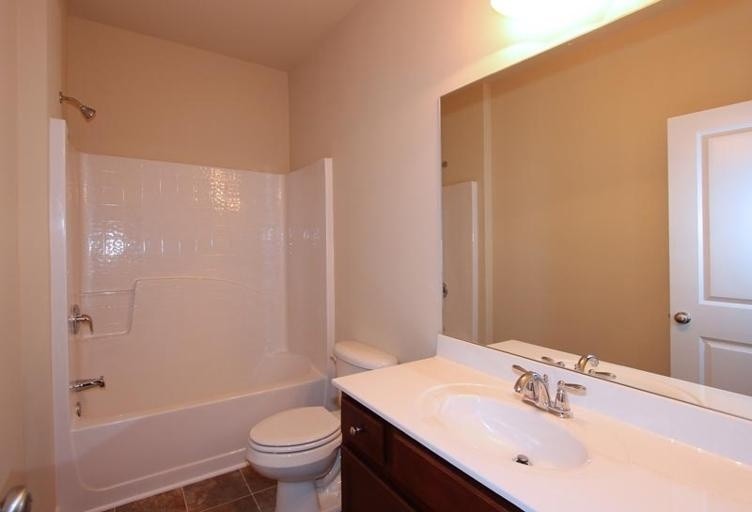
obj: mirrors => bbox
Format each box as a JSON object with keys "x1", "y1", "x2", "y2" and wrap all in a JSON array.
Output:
[{"x1": 439, "y1": 0, "x2": 752, "y2": 423}]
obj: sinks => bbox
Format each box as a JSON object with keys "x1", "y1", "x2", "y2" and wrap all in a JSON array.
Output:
[
  {"x1": 418, "y1": 382, "x2": 590, "y2": 471},
  {"x1": 560, "y1": 361, "x2": 702, "y2": 406}
]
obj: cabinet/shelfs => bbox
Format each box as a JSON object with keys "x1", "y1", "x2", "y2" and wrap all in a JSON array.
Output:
[{"x1": 341, "y1": 391, "x2": 527, "y2": 512}]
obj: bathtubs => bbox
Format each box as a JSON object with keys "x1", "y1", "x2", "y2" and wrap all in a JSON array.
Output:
[{"x1": 70, "y1": 353, "x2": 327, "y2": 511}]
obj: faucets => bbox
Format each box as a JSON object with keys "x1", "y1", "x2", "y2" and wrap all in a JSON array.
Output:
[
  {"x1": 574, "y1": 354, "x2": 599, "y2": 373},
  {"x1": 75, "y1": 376, "x2": 105, "y2": 392},
  {"x1": 514, "y1": 372, "x2": 550, "y2": 409}
]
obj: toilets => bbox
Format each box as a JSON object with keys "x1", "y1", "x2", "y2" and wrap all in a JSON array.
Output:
[{"x1": 244, "y1": 338, "x2": 399, "y2": 511}]
